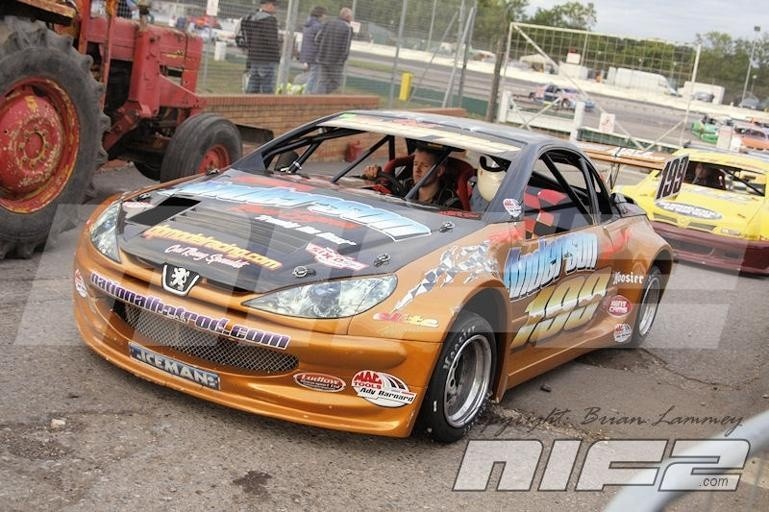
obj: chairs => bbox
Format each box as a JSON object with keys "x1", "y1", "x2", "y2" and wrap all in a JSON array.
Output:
[
  {"x1": 380, "y1": 156, "x2": 474, "y2": 211},
  {"x1": 693, "y1": 167, "x2": 725, "y2": 187},
  {"x1": 517, "y1": 186, "x2": 576, "y2": 240}
]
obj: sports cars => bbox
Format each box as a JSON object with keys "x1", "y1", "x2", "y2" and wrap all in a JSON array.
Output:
[
  {"x1": 71, "y1": 107, "x2": 674, "y2": 446},
  {"x1": 529, "y1": 82, "x2": 595, "y2": 113},
  {"x1": 692, "y1": 113, "x2": 768, "y2": 153},
  {"x1": 607, "y1": 148, "x2": 768, "y2": 278}
]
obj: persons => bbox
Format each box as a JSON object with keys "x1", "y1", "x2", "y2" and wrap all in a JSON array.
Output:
[
  {"x1": 246, "y1": 0, "x2": 281, "y2": 93},
  {"x1": 300, "y1": 5, "x2": 328, "y2": 94},
  {"x1": 363, "y1": 145, "x2": 464, "y2": 210},
  {"x1": 313, "y1": 7, "x2": 353, "y2": 95},
  {"x1": 685, "y1": 163, "x2": 717, "y2": 187}
]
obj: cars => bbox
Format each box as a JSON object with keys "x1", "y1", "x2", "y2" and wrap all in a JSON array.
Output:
[
  {"x1": 691, "y1": 92, "x2": 714, "y2": 104},
  {"x1": 739, "y1": 96, "x2": 768, "y2": 111}
]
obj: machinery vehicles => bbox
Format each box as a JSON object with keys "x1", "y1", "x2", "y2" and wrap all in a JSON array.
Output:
[{"x1": 0, "y1": 0, "x2": 244, "y2": 268}]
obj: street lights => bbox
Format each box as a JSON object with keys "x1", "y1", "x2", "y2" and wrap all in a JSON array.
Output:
[{"x1": 740, "y1": 25, "x2": 763, "y2": 101}]
